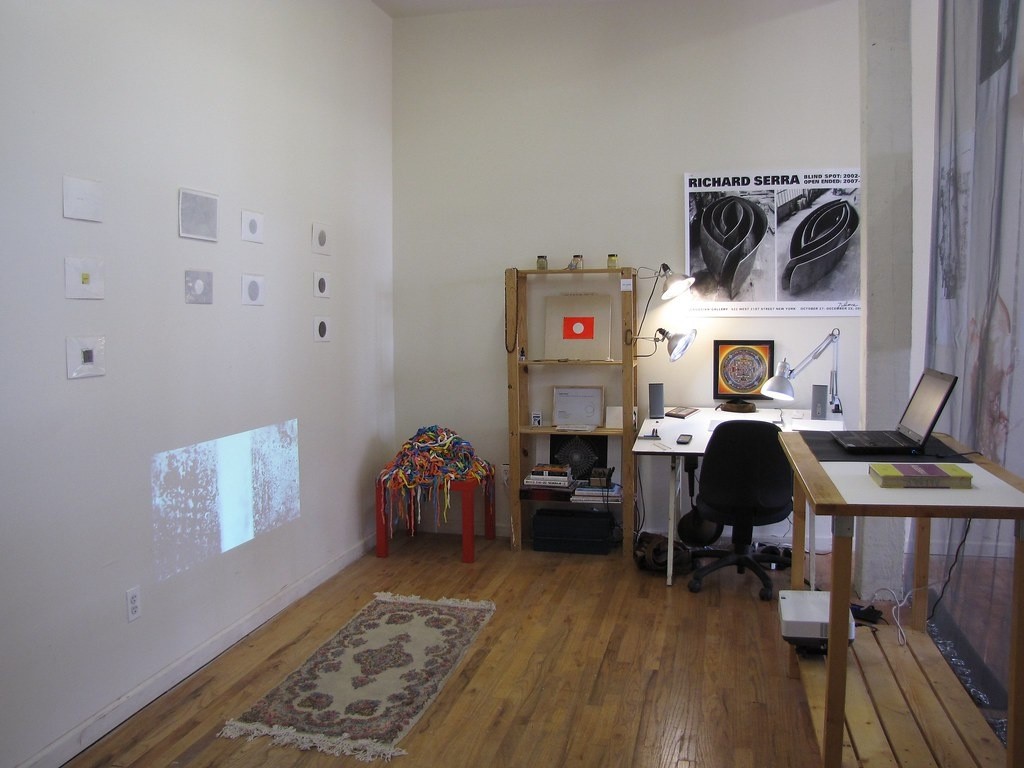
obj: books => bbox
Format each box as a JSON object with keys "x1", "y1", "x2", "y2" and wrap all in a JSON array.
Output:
[
  {"x1": 571, "y1": 477, "x2": 623, "y2": 504},
  {"x1": 868, "y1": 462, "x2": 973, "y2": 489},
  {"x1": 524, "y1": 462, "x2": 576, "y2": 489}
]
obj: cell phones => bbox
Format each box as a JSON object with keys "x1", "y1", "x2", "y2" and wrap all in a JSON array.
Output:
[{"x1": 677, "y1": 434, "x2": 692, "y2": 445}]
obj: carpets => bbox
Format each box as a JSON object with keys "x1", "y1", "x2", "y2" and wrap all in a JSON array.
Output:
[{"x1": 218, "y1": 590, "x2": 495, "y2": 762}]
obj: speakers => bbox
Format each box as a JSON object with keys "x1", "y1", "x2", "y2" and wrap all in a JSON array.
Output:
[
  {"x1": 811, "y1": 385, "x2": 828, "y2": 420},
  {"x1": 649, "y1": 383, "x2": 664, "y2": 419}
]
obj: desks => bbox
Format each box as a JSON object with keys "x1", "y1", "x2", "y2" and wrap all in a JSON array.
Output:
[
  {"x1": 631, "y1": 406, "x2": 844, "y2": 586},
  {"x1": 778, "y1": 431, "x2": 1024, "y2": 768}
]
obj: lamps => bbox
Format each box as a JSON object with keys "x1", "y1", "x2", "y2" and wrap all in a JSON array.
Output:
[
  {"x1": 635, "y1": 327, "x2": 701, "y2": 362},
  {"x1": 638, "y1": 262, "x2": 698, "y2": 338},
  {"x1": 762, "y1": 327, "x2": 842, "y2": 415}
]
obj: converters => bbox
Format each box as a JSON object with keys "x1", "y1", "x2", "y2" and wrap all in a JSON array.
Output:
[{"x1": 850, "y1": 604, "x2": 883, "y2": 624}]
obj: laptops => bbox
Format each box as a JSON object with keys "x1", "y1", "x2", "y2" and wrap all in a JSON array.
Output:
[{"x1": 830, "y1": 367, "x2": 958, "y2": 453}]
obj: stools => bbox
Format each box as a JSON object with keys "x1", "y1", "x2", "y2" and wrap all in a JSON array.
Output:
[{"x1": 375, "y1": 465, "x2": 497, "y2": 564}]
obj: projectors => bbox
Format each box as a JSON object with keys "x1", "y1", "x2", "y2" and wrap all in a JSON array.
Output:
[{"x1": 777, "y1": 590, "x2": 855, "y2": 645}]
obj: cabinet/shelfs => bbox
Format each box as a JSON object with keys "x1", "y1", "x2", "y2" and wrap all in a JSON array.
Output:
[{"x1": 505, "y1": 267, "x2": 637, "y2": 559}]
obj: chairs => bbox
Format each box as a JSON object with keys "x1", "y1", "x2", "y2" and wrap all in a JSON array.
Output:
[{"x1": 687, "y1": 420, "x2": 794, "y2": 600}]
